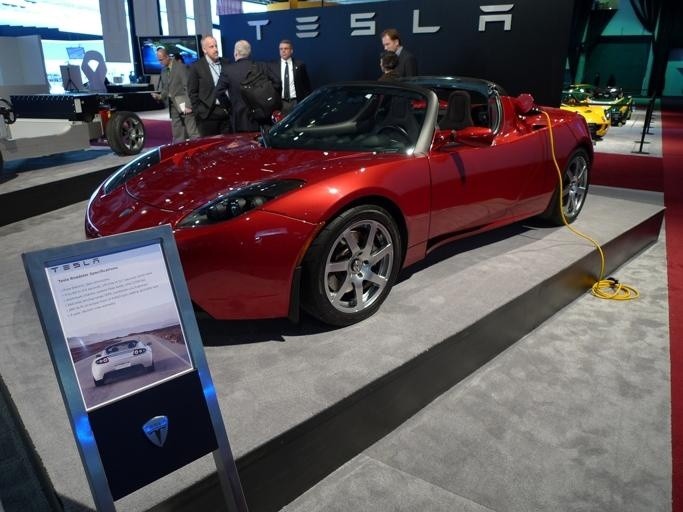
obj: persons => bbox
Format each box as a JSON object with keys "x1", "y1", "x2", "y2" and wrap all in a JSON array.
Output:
[
  {"x1": 377, "y1": 28, "x2": 416, "y2": 81},
  {"x1": 150, "y1": 34, "x2": 311, "y2": 144}
]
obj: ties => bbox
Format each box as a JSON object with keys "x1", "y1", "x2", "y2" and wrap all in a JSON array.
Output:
[{"x1": 284, "y1": 62, "x2": 290, "y2": 101}]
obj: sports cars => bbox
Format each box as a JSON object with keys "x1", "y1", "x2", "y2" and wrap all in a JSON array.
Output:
[
  {"x1": 89, "y1": 339, "x2": 154, "y2": 387},
  {"x1": 85, "y1": 76, "x2": 594, "y2": 327},
  {"x1": 559, "y1": 83, "x2": 633, "y2": 140}
]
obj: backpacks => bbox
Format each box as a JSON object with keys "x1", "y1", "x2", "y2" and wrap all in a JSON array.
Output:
[{"x1": 239, "y1": 60, "x2": 283, "y2": 125}]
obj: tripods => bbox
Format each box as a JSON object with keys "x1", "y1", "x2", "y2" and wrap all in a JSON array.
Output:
[{"x1": 65, "y1": 68, "x2": 79, "y2": 92}]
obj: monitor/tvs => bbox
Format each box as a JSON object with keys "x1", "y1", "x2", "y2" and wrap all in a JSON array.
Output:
[{"x1": 137, "y1": 34, "x2": 200, "y2": 76}]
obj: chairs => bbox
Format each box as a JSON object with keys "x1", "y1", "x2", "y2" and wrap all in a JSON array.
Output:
[{"x1": 366, "y1": 91, "x2": 476, "y2": 144}]
